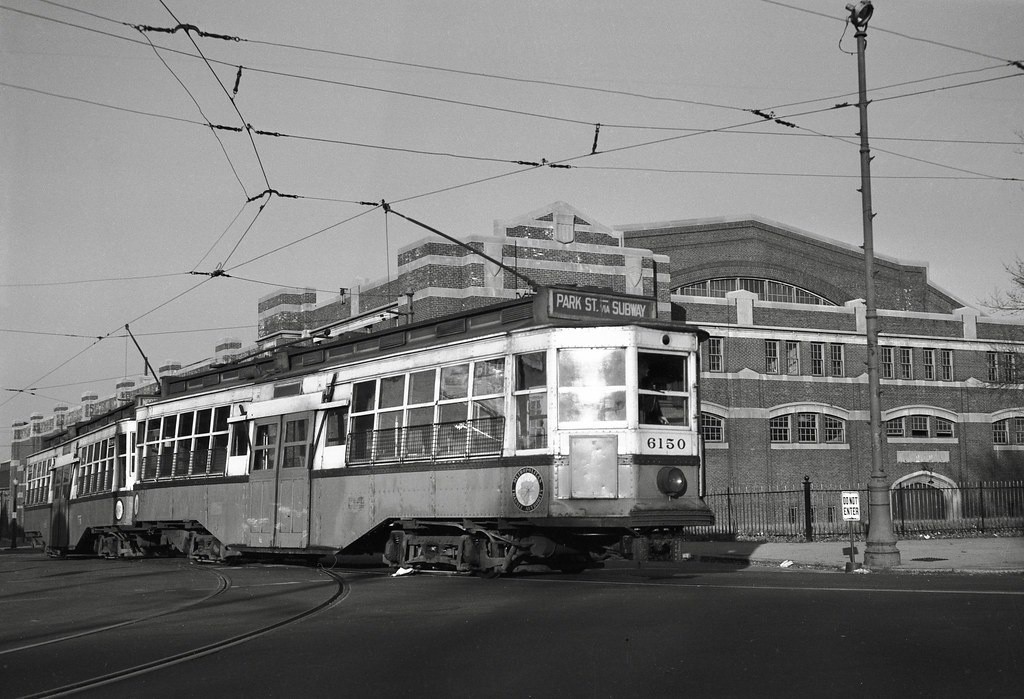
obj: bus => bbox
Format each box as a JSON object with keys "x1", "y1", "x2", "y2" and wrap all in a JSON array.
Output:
[{"x1": 23, "y1": 197, "x2": 716, "y2": 581}]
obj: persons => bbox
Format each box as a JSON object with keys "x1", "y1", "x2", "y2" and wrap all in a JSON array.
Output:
[{"x1": 638, "y1": 359, "x2": 669, "y2": 424}]
